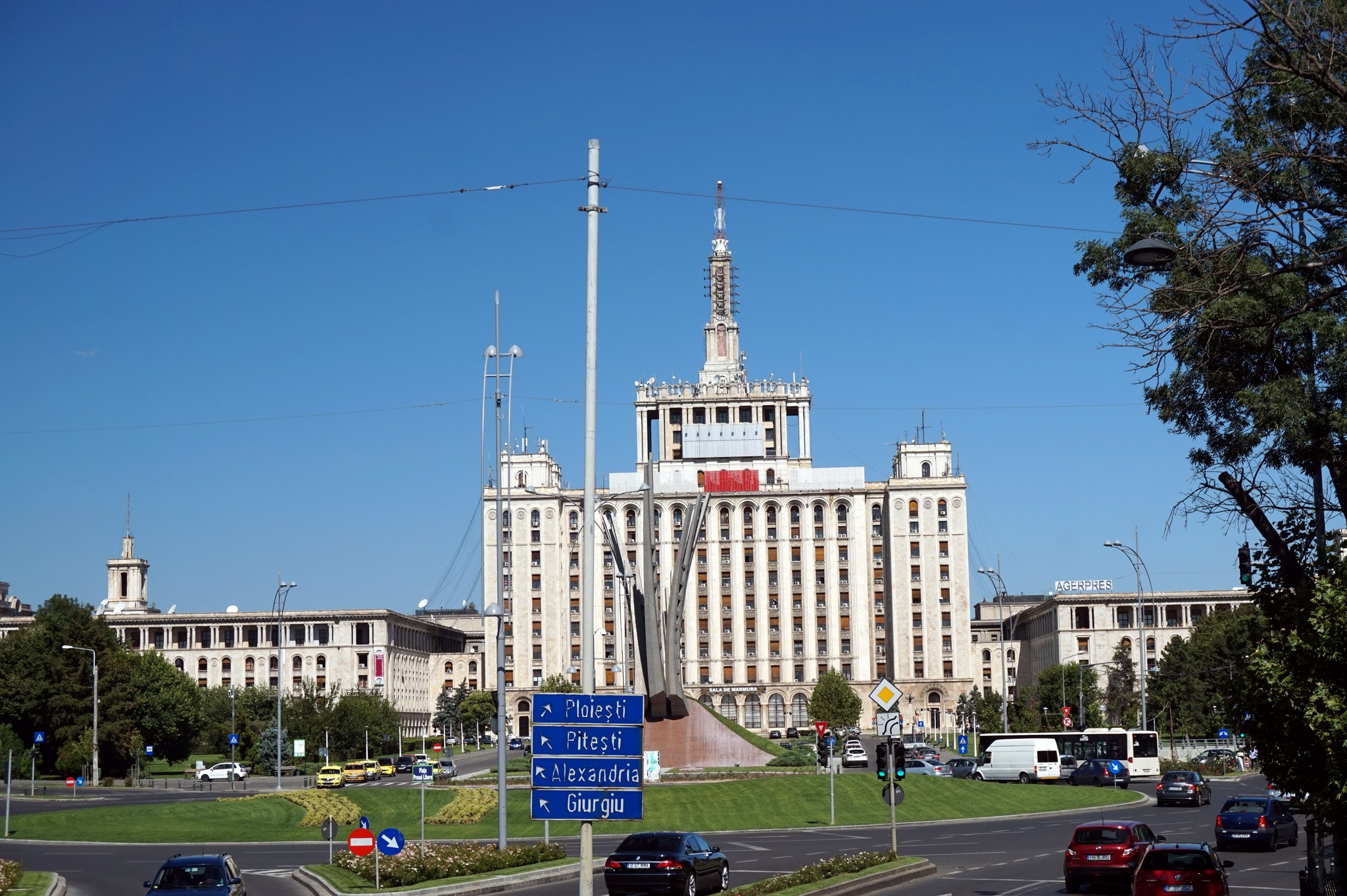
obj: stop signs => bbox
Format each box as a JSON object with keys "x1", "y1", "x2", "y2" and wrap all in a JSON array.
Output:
[
  {"x1": 66, "y1": 777, "x2": 75, "y2": 787},
  {"x1": 433, "y1": 744, "x2": 442, "y2": 752},
  {"x1": 348, "y1": 829, "x2": 376, "y2": 856}
]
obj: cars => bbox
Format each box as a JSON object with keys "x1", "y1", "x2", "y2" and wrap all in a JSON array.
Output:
[
  {"x1": 764, "y1": 725, "x2": 978, "y2": 781},
  {"x1": 1191, "y1": 749, "x2": 1240, "y2": 770},
  {"x1": 414, "y1": 733, "x2": 532, "y2": 757},
  {"x1": 1156, "y1": 770, "x2": 1210, "y2": 807},
  {"x1": 342, "y1": 763, "x2": 368, "y2": 783},
  {"x1": 358, "y1": 753, "x2": 461, "y2": 781},
  {"x1": 603, "y1": 829, "x2": 730, "y2": 896},
  {"x1": 316, "y1": 766, "x2": 346, "y2": 788},
  {"x1": 1069, "y1": 760, "x2": 1130, "y2": 790}
]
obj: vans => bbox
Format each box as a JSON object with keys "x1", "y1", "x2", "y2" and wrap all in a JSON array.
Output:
[
  {"x1": 971, "y1": 738, "x2": 1062, "y2": 786},
  {"x1": 1052, "y1": 754, "x2": 1078, "y2": 784}
]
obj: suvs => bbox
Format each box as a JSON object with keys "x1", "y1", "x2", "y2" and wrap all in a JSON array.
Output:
[
  {"x1": 1214, "y1": 794, "x2": 1299, "y2": 853},
  {"x1": 196, "y1": 762, "x2": 248, "y2": 783},
  {"x1": 1064, "y1": 821, "x2": 1167, "y2": 892},
  {"x1": 1131, "y1": 838, "x2": 1235, "y2": 896},
  {"x1": 1267, "y1": 779, "x2": 1322, "y2": 812},
  {"x1": 142, "y1": 853, "x2": 248, "y2": 896}
]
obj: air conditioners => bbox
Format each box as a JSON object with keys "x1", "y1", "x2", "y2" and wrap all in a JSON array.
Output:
[
  {"x1": 445, "y1": 669, "x2": 474, "y2": 692},
  {"x1": 501, "y1": 509, "x2": 953, "y2": 687},
  {"x1": 246, "y1": 663, "x2": 365, "y2": 691}
]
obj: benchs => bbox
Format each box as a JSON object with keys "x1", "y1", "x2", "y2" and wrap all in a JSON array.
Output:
[
  {"x1": 273, "y1": 766, "x2": 296, "y2": 777},
  {"x1": 464, "y1": 743, "x2": 498, "y2": 754},
  {"x1": 923, "y1": 736, "x2": 948, "y2": 752},
  {"x1": 182, "y1": 769, "x2": 205, "y2": 779},
  {"x1": 245, "y1": 767, "x2": 252, "y2": 778}
]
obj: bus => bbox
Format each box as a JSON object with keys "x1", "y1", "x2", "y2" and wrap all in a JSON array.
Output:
[{"x1": 979, "y1": 727, "x2": 1160, "y2": 777}]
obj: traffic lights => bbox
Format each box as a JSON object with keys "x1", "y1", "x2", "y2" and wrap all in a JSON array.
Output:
[
  {"x1": 1240, "y1": 728, "x2": 1245, "y2": 738},
  {"x1": 1239, "y1": 551, "x2": 1252, "y2": 585}
]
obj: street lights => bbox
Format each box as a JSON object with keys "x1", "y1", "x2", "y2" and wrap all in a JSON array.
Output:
[
  {"x1": 923, "y1": 707, "x2": 977, "y2": 757},
  {"x1": 905, "y1": 695, "x2": 916, "y2": 732},
  {"x1": 62, "y1": 645, "x2": 99, "y2": 787},
  {"x1": 325, "y1": 726, "x2": 329, "y2": 766},
  {"x1": 565, "y1": 657, "x2": 621, "y2": 692},
  {"x1": 480, "y1": 343, "x2": 522, "y2": 850},
  {"x1": 266, "y1": 582, "x2": 298, "y2": 790},
  {"x1": 1103, "y1": 539, "x2": 1157, "y2": 731},
  {"x1": 365, "y1": 717, "x2": 494, "y2": 761},
  {"x1": 977, "y1": 567, "x2": 1015, "y2": 735},
  {"x1": 1062, "y1": 650, "x2": 1084, "y2": 731}
]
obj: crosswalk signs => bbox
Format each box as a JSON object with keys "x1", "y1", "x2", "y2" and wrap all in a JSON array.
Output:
[
  {"x1": 34, "y1": 732, "x2": 45, "y2": 742},
  {"x1": 229, "y1": 734, "x2": 239, "y2": 745}
]
